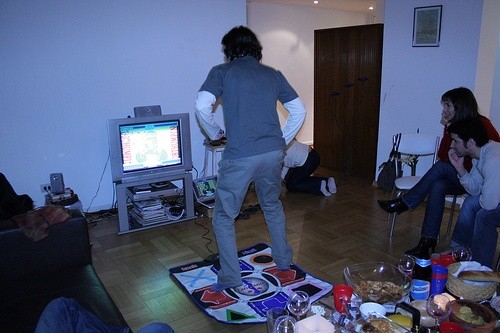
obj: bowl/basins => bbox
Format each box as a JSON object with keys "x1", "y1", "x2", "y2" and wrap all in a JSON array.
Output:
[
  {"x1": 344, "y1": 262, "x2": 414, "y2": 305},
  {"x1": 359, "y1": 302, "x2": 387, "y2": 321},
  {"x1": 444, "y1": 299, "x2": 495, "y2": 333},
  {"x1": 409, "y1": 300, "x2": 439, "y2": 327}
]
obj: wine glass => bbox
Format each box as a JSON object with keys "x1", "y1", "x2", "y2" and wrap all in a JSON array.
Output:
[
  {"x1": 426, "y1": 294, "x2": 452, "y2": 328},
  {"x1": 286, "y1": 291, "x2": 310, "y2": 321},
  {"x1": 398, "y1": 254, "x2": 416, "y2": 284}
]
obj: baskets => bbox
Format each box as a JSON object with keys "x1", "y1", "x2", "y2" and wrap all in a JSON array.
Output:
[{"x1": 446, "y1": 261, "x2": 497, "y2": 301}]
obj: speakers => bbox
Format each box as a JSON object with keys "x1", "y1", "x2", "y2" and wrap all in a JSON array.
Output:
[
  {"x1": 50, "y1": 173, "x2": 64, "y2": 194},
  {"x1": 133, "y1": 105, "x2": 162, "y2": 118}
]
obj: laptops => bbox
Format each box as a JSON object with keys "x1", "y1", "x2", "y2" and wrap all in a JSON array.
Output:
[{"x1": 192, "y1": 175, "x2": 218, "y2": 208}]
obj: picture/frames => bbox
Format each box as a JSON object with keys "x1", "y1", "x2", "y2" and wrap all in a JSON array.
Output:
[{"x1": 411, "y1": 4, "x2": 443, "y2": 47}]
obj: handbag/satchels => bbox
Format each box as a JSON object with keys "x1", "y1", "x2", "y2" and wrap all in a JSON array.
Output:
[{"x1": 376, "y1": 133, "x2": 403, "y2": 190}]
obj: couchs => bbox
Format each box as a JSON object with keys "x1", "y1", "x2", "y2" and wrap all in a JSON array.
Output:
[{"x1": 0, "y1": 223, "x2": 133, "y2": 333}]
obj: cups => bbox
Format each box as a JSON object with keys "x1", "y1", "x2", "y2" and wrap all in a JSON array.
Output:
[
  {"x1": 410, "y1": 279, "x2": 431, "y2": 301},
  {"x1": 451, "y1": 245, "x2": 472, "y2": 263},
  {"x1": 333, "y1": 284, "x2": 353, "y2": 314},
  {"x1": 266, "y1": 307, "x2": 299, "y2": 333},
  {"x1": 430, "y1": 264, "x2": 449, "y2": 297}
]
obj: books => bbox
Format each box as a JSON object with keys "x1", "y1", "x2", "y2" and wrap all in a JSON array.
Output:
[{"x1": 130, "y1": 199, "x2": 171, "y2": 226}]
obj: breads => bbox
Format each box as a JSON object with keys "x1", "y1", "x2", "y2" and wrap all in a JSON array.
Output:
[
  {"x1": 458, "y1": 270, "x2": 500, "y2": 282},
  {"x1": 361, "y1": 319, "x2": 392, "y2": 333}
]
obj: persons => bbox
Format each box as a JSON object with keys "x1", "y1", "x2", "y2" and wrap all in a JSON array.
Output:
[
  {"x1": 377, "y1": 87, "x2": 500, "y2": 260},
  {"x1": 195, "y1": 25, "x2": 307, "y2": 292},
  {"x1": 280, "y1": 140, "x2": 337, "y2": 197},
  {"x1": 34, "y1": 298, "x2": 176, "y2": 333},
  {"x1": 447, "y1": 118, "x2": 500, "y2": 269}
]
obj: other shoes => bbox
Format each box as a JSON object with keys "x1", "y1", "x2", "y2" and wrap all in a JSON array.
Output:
[{"x1": 443, "y1": 245, "x2": 452, "y2": 251}]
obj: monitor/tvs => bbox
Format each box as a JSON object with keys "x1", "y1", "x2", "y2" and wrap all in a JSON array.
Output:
[{"x1": 107, "y1": 113, "x2": 193, "y2": 184}]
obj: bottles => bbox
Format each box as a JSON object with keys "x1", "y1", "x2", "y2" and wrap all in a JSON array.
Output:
[{"x1": 413, "y1": 237, "x2": 432, "y2": 281}]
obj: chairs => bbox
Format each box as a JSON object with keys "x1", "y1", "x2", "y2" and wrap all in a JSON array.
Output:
[{"x1": 390, "y1": 133, "x2": 500, "y2": 275}]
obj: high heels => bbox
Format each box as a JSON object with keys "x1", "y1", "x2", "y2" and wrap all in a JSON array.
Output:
[
  {"x1": 404, "y1": 237, "x2": 437, "y2": 260},
  {"x1": 377, "y1": 197, "x2": 408, "y2": 216}
]
